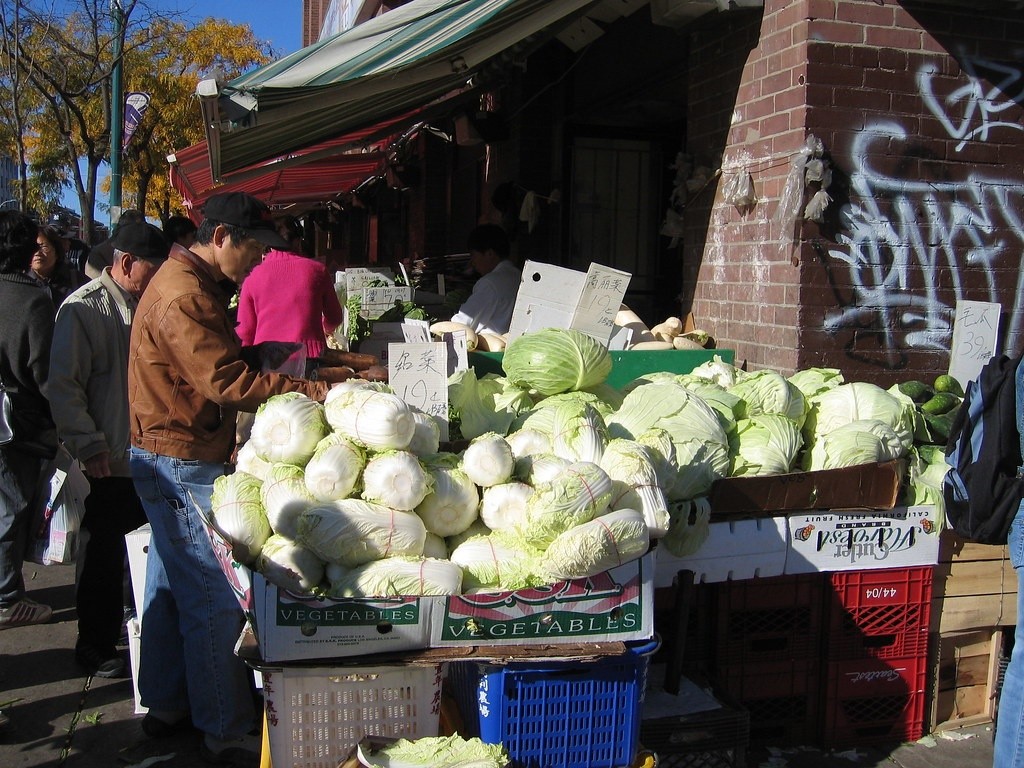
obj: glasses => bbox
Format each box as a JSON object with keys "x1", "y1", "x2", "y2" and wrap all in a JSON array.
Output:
[{"x1": 39, "y1": 244, "x2": 52, "y2": 252}]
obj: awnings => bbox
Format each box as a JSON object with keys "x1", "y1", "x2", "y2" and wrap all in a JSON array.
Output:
[
  {"x1": 169, "y1": 105, "x2": 426, "y2": 227},
  {"x1": 196, "y1": 0, "x2": 599, "y2": 183}
]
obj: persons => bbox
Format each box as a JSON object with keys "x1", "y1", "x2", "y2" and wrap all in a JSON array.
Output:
[
  {"x1": 450, "y1": 224, "x2": 521, "y2": 332},
  {"x1": 233, "y1": 213, "x2": 343, "y2": 379},
  {"x1": 0, "y1": 192, "x2": 339, "y2": 768}
]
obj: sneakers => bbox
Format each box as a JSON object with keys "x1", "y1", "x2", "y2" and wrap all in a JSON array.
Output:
[
  {"x1": 76, "y1": 642, "x2": 126, "y2": 678},
  {"x1": 0, "y1": 597, "x2": 53, "y2": 630}
]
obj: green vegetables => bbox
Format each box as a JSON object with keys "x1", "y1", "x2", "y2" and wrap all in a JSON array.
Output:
[{"x1": 346, "y1": 278, "x2": 388, "y2": 336}]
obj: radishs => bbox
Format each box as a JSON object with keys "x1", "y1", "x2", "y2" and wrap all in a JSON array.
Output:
[{"x1": 428, "y1": 304, "x2": 709, "y2": 353}]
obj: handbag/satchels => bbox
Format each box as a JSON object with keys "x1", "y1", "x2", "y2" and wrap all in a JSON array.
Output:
[
  {"x1": 23, "y1": 459, "x2": 80, "y2": 567},
  {"x1": 1, "y1": 389, "x2": 60, "y2": 461}
]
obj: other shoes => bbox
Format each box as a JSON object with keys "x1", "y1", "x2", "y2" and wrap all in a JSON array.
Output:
[
  {"x1": 142, "y1": 706, "x2": 197, "y2": 738},
  {"x1": 202, "y1": 725, "x2": 260, "y2": 764}
]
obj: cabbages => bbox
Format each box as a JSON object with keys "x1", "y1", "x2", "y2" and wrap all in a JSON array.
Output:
[
  {"x1": 355, "y1": 732, "x2": 510, "y2": 768},
  {"x1": 211, "y1": 328, "x2": 951, "y2": 597}
]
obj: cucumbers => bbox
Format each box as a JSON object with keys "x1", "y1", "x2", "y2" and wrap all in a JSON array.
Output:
[
  {"x1": 377, "y1": 303, "x2": 423, "y2": 322},
  {"x1": 897, "y1": 375, "x2": 969, "y2": 462}
]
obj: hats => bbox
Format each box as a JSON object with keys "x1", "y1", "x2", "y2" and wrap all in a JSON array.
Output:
[
  {"x1": 110, "y1": 221, "x2": 171, "y2": 259},
  {"x1": 49, "y1": 224, "x2": 75, "y2": 238},
  {"x1": 166, "y1": 217, "x2": 197, "y2": 241},
  {"x1": 47, "y1": 214, "x2": 67, "y2": 225},
  {"x1": 204, "y1": 191, "x2": 290, "y2": 249}
]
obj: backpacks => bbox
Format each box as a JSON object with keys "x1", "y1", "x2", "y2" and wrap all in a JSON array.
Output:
[{"x1": 941, "y1": 349, "x2": 1024, "y2": 547}]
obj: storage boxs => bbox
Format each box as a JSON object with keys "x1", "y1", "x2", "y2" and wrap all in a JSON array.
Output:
[{"x1": 123, "y1": 268, "x2": 1020, "y2": 768}]
